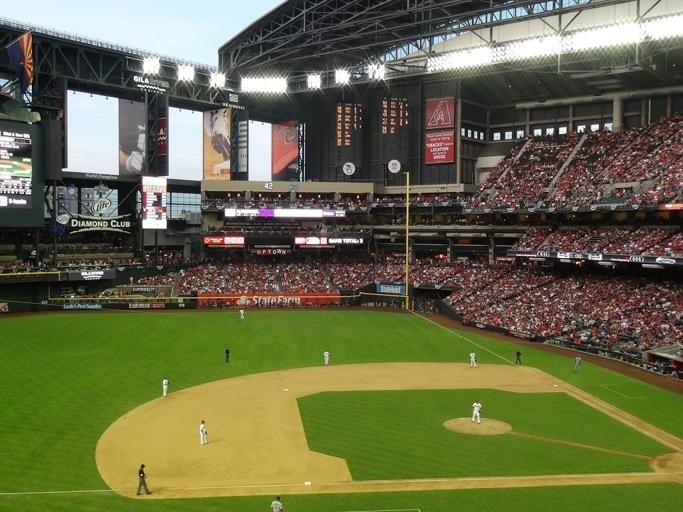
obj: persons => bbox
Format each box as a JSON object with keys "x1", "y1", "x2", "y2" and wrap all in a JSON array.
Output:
[
  {"x1": 223, "y1": 346, "x2": 231, "y2": 366},
  {"x1": 238, "y1": 308, "x2": 245, "y2": 320},
  {"x1": 1, "y1": 242, "x2": 405, "y2": 313},
  {"x1": 161, "y1": 376, "x2": 169, "y2": 397},
  {"x1": 199, "y1": 420, "x2": 208, "y2": 445},
  {"x1": 408, "y1": 244, "x2": 682, "y2": 312},
  {"x1": 200, "y1": 195, "x2": 466, "y2": 235},
  {"x1": 135, "y1": 464, "x2": 151, "y2": 495},
  {"x1": 462, "y1": 110, "x2": 681, "y2": 243},
  {"x1": 470, "y1": 400, "x2": 482, "y2": 424},
  {"x1": 456, "y1": 313, "x2": 682, "y2": 382},
  {"x1": 321, "y1": 349, "x2": 330, "y2": 365},
  {"x1": 118, "y1": 106, "x2": 146, "y2": 174},
  {"x1": 269, "y1": 496, "x2": 282, "y2": 512}
]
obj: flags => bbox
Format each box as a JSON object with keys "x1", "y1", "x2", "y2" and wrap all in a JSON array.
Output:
[{"x1": 5, "y1": 31, "x2": 33, "y2": 95}]
